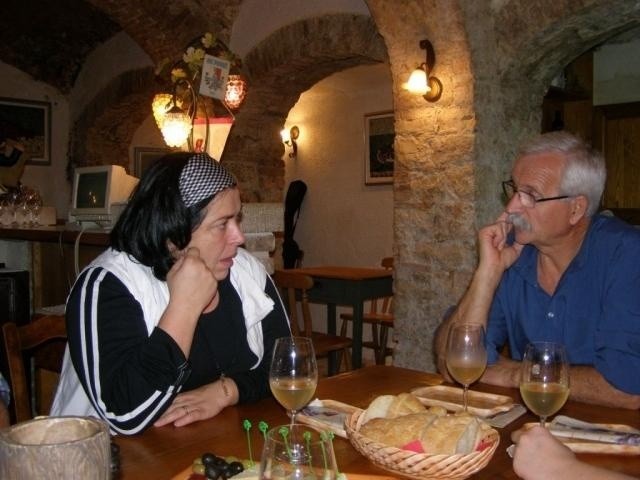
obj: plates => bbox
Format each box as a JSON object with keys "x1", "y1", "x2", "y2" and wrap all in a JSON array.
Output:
[
  {"x1": 512, "y1": 420, "x2": 640, "y2": 457},
  {"x1": 410, "y1": 383, "x2": 513, "y2": 418},
  {"x1": 290, "y1": 398, "x2": 366, "y2": 440}
]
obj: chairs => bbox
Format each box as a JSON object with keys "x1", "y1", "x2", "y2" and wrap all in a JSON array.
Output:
[
  {"x1": 334, "y1": 254, "x2": 397, "y2": 372},
  {"x1": 2, "y1": 307, "x2": 94, "y2": 432},
  {"x1": 270, "y1": 264, "x2": 353, "y2": 383}
]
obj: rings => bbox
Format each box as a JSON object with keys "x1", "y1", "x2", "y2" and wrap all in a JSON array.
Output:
[{"x1": 180, "y1": 403, "x2": 194, "y2": 417}]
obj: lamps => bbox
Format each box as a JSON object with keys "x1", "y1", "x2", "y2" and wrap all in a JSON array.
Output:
[
  {"x1": 152, "y1": 33, "x2": 248, "y2": 148},
  {"x1": 397, "y1": 38, "x2": 447, "y2": 106},
  {"x1": 278, "y1": 120, "x2": 304, "y2": 159}
]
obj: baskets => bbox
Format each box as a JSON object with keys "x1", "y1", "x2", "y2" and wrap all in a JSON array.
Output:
[{"x1": 345, "y1": 410, "x2": 500, "y2": 479}]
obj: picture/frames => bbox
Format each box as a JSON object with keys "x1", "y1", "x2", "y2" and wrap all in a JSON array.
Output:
[
  {"x1": 362, "y1": 107, "x2": 400, "y2": 188},
  {"x1": 0, "y1": 96, "x2": 58, "y2": 168}
]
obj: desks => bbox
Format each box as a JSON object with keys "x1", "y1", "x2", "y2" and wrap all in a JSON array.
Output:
[
  {"x1": 279, "y1": 264, "x2": 394, "y2": 378},
  {"x1": 0, "y1": 213, "x2": 292, "y2": 410}
]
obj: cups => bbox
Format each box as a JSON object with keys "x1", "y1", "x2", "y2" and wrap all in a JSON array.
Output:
[
  {"x1": 0, "y1": 416, "x2": 113, "y2": 479},
  {"x1": 255, "y1": 425, "x2": 341, "y2": 480}
]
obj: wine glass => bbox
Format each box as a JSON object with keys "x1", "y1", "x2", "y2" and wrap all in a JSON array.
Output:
[
  {"x1": 446, "y1": 321, "x2": 490, "y2": 416},
  {"x1": 518, "y1": 341, "x2": 573, "y2": 428},
  {"x1": 7, "y1": 187, "x2": 44, "y2": 228},
  {"x1": 268, "y1": 336, "x2": 320, "y2": 464}
]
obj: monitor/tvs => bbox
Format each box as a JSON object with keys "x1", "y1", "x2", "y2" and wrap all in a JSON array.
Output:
[{"x1": 71, "y1": 165, "x2": 140, "y2": 230}]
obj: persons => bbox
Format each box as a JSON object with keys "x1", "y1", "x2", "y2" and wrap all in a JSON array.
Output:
[
  {"x1": 431, "y1": 130, "x2": 640, "y2": 413},
  {"x1": 509, "y1": 425, "x2": 640, "y2": 479},
  {"x1": 49, "y1": 151, "x2": 295, "y2": 438}
]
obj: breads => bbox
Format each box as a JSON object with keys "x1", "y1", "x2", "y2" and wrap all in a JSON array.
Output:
[{"x1": 348, "y1": 391, "x2": 499, "y2": 458}]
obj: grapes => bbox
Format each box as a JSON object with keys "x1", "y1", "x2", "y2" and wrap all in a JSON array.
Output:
[{"x1": 192, "y1": 451, "x2": 243, "y2": 479}]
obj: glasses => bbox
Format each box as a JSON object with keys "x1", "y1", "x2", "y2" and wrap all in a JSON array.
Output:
[{"x1": 502, "y1": 180, "x2": 568, "y2": 208}]
